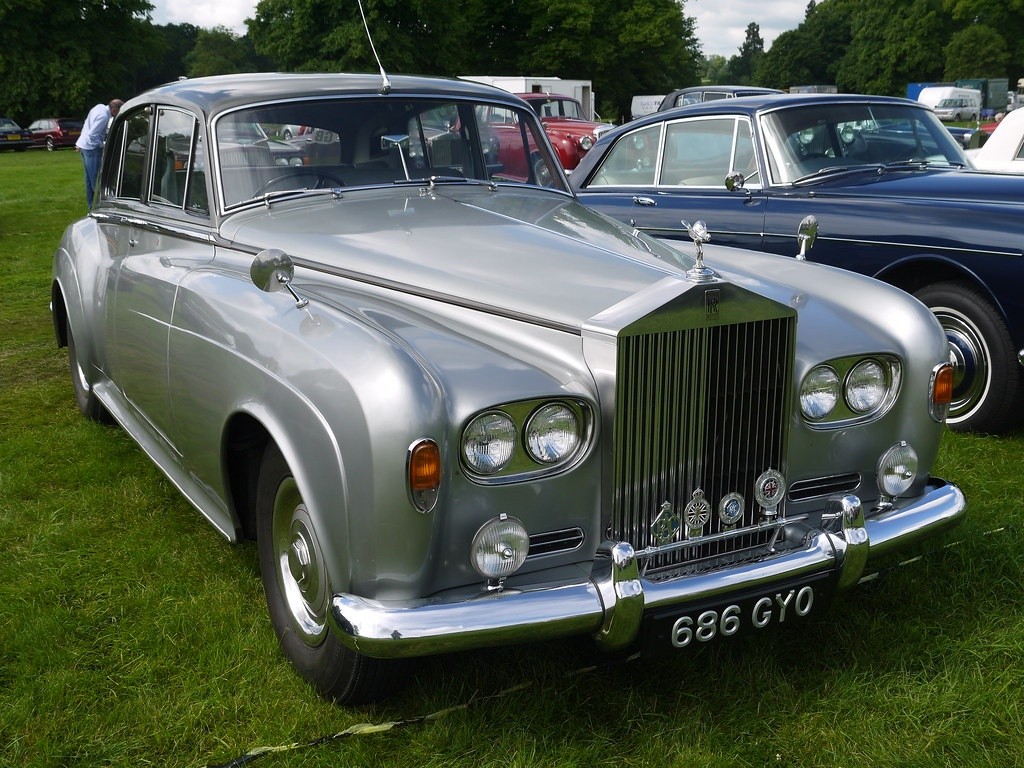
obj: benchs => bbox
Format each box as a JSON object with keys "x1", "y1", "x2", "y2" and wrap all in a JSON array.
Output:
[
  {"x1": 594, "y1": 168, "x2": 750, "y2": 185},
  {"x1": 174, "y1": 165, "x2": 463, "y2": 213}
]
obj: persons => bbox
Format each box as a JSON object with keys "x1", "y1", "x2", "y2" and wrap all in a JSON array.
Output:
[{"x1": 74, "y1": 98, "x2": 125, "y2": 211}]
